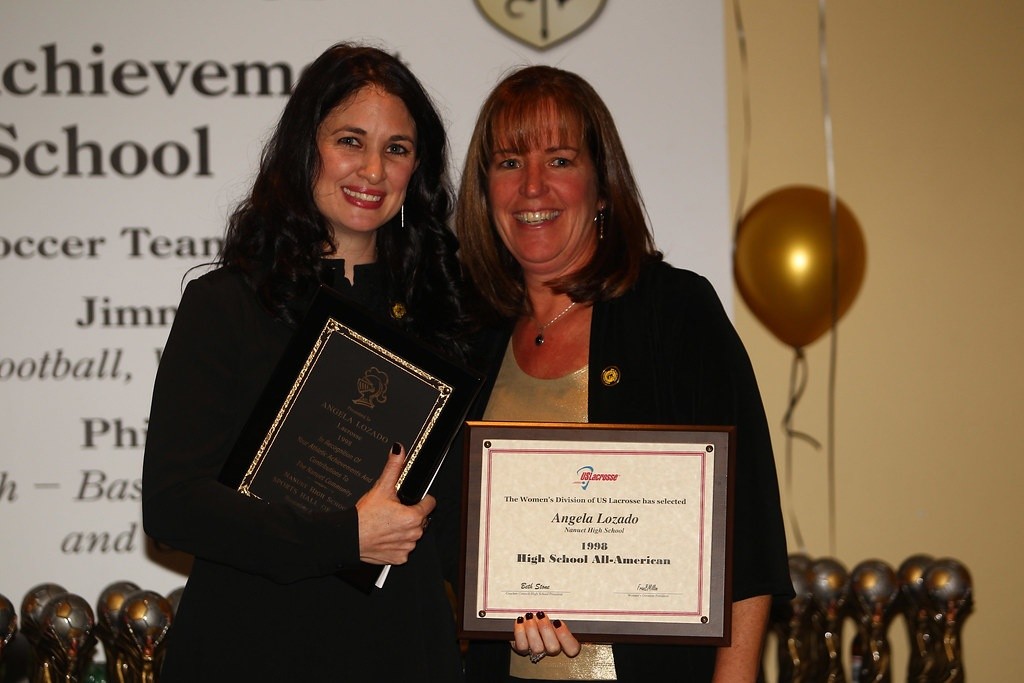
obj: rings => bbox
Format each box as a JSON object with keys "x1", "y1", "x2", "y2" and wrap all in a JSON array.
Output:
[{"x1": 529, "y1": 648, "x2": 547, "y2": 663}]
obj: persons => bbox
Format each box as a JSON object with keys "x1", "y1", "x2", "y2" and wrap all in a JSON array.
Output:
[
  {"x1": 435, "y1": 66, "x2": 796, "y2": 683},
  {"x1": 141, "y1": 45, "x2": 484, "y2": 683}
]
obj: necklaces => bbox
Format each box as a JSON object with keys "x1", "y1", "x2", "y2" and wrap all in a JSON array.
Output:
[{"x1": 532, "y1": 302, "x2": 576, "y2": 345}]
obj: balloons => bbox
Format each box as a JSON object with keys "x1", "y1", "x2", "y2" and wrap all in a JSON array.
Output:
[{"x1": 735, "y1": 186, "x2": 867, "y2": 360}]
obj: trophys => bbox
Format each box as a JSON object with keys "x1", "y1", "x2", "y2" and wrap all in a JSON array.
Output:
[
  {"x1": 0, "y1": 581, "x2": 185, "y2": 683},
  {"x1": 789, "y1": 553, "x2": 974, "y2": 683}
]
